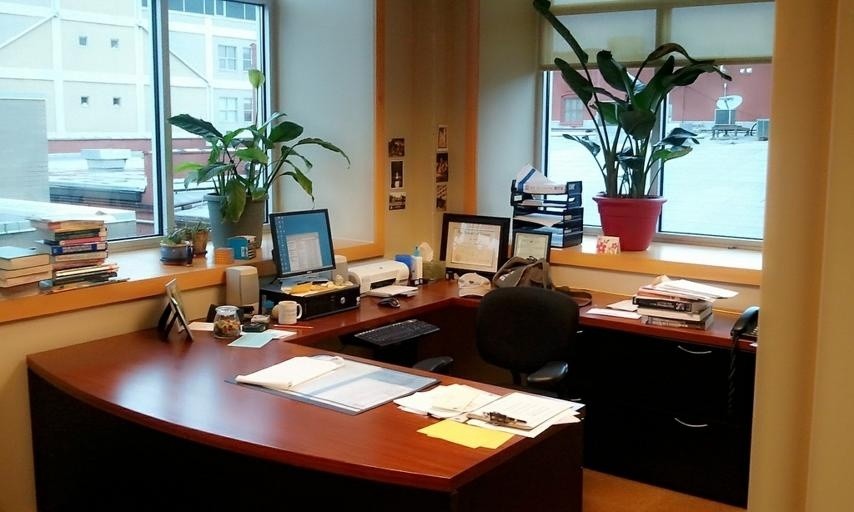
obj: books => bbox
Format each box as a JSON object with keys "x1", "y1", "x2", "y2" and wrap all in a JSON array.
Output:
[
  {"x1": 632, "y1": 272, "x2": 741, "y2": 332},
  {"x1": 234, "y1": 356, "x2": 347, "y2": 392},
  {"x1": 0, "y1": 215, "x2": 119, "y2": 290}
]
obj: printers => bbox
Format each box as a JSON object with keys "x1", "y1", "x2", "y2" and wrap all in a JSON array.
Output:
[{"x1": 348, "y1": 260, "x2": 419, "y2": 299}]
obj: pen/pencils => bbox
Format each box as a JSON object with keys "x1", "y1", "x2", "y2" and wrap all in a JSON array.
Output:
[{"x1": 272, "y1": 323, "x2": 317, "y2": 330}]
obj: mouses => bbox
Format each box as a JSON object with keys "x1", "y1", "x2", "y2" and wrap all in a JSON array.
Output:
[{"x1": 378, "y1": 299, "x2": 400, "y2": 307}]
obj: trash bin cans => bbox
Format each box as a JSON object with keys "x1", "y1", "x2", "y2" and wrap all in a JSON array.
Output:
[{"x1": 757, "y1": 119, "x2": 769, "y2": 141}]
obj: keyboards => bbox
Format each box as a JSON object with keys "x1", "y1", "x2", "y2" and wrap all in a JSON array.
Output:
[{"x1": 354, "y1": 318, "x2": 440, "y2": 346}]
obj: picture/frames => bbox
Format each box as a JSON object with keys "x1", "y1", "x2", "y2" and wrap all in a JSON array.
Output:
[
  {"x1": 511, "y1": 229, "x2": 552, "y2": 263},
  {"x1": 440, "y1": 212, "x2": 511, "y2": 279}
]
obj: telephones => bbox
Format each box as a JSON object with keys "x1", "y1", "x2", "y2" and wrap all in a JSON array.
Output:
[{"x1": 729, "y1": 305, "x2": 759, "y2": 342}]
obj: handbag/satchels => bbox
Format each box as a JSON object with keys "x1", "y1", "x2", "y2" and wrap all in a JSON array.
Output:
[{"x1": 493, "y1": 256, "x2": 592, "y2": 307}]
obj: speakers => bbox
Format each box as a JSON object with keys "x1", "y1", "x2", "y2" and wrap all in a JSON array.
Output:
[
  {"x1": 225, "y1": 266, "x2": 260, "y2": 317},
  {"x1": 332, "y1": 254, "x2": 348, "y2": 282}
]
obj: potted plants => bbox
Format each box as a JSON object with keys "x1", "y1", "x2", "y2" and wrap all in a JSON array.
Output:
[
  {"x1": 160, "y1": 222, "x2": 194, "y2": 265},
  {"x1": 166, "y1": 69, "x2": 351, "y2": 253},
  {"x1": 184, "y1": 219, "x2": 213, "y2": 258},
  {"x1": 532, "y1": 0, "x2": 732, "y2": 251}
]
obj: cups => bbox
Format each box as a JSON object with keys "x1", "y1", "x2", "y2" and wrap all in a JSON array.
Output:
[{"x1": 279, "y1": 300, "x2": 304, "y2": 324}]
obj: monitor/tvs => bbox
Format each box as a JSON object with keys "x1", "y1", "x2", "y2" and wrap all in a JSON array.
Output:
[{"x1": 269, "y1": 208, "x2": 336, "y2": 285}]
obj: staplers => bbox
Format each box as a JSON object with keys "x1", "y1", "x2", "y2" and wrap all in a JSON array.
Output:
[{"x1": 239, "y1": 306, "x2": 255, "y2": 325}]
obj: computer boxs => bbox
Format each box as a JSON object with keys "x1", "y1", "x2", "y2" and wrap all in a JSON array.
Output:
[{"x1": 261, "y1": 278, "x2": 361, "y2": 319}]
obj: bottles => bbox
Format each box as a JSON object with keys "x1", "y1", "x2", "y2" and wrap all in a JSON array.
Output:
[
  {"x1": 395, "y1": 254, "x2": 412, "y2": 285},
  {"x1": 185, "y1": 241, "x2": 194, "y2": 267},
  {"x1": 213, "y1": 305, "x2": 242, "y2": 338}
]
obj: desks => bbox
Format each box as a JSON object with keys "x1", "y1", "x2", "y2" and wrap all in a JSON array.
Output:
[{"x1": 25, "y1": 279, "x2": 760, "y2": 512}]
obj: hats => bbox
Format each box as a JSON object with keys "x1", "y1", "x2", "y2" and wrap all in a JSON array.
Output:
[{"x1": 458, "y1": 273, "x2": 491, "y2": 297}]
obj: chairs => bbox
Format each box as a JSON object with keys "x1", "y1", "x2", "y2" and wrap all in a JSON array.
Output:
[{"x1": 412, "y1": 287, "x2": 585, "y2": 398}]
